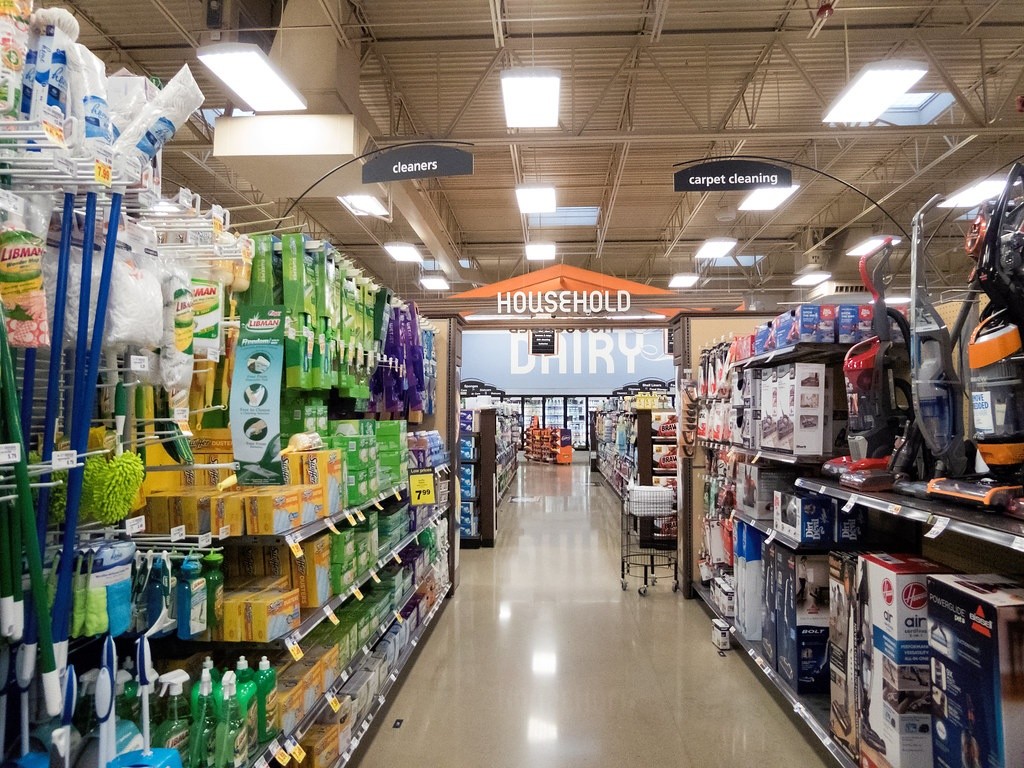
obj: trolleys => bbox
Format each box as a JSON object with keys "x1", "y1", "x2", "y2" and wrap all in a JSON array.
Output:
[{"x1": 620, "y1": 477, "x2": 679, "y2": 597}]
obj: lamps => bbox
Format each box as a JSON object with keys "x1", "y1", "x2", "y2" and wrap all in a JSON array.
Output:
[
  {"x1": 868, "y1": 292, "x2": 912, "y2": 304},
  {"x1": 196, "y1": 0, "x2": 1024, "y2": 290}
]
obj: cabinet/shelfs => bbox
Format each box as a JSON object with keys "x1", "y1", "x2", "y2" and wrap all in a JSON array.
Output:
[
  {"x1": 458, "y1": 378, "x2": 518, "y2": 550},
  {"x1": 693, "y1": 337, "x2": 1024, "y2": 768},
  {"x1": 595, "y1": 379, "x2": 678, "y2": 551},
  {"x1": 0, "y1": 112, "x2": 452, "y2": 768},
  {"x1": 523, "y1": 428, "x2": 573, "y2": 465}
]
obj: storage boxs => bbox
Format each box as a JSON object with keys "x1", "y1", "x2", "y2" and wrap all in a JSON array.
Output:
[
  {"x1": 95, "y1": 232, "x2": 426, "y2": 768},
  {"x1": 711, "y1": 304, "x2": 1024, "y2": 768}
]
